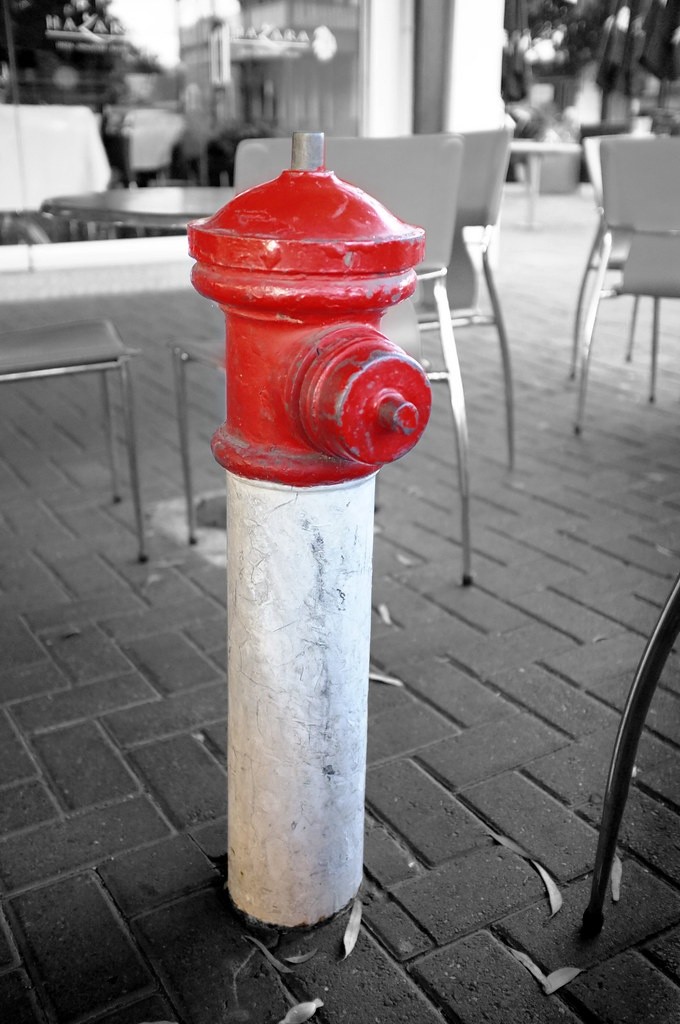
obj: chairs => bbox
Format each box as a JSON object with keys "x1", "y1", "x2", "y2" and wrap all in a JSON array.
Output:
[
  {"x1": 165, "y1": 131, "x2": 475, "y2": 591},
  {"x1": 567, "y1": 134, "x2": 679, "y2": 437},
  {"x1": 1, "y1": 319, "x2": 149, "y2": 567},
  {"x1": 567, "y1": 130, "x2": 641, "y2": 380},
  {"x1": 406, "y1": 123, "x2": 515, "y2": 474}
]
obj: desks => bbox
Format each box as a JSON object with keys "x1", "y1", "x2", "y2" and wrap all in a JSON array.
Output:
[
  {"x1": 44, "y1": 185, "x2": 242, "y2": 233},
  {"x1": 512, "y1": 142, "x2": 579, "y2": 230}
]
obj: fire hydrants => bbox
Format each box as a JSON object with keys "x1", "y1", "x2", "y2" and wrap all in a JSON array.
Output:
[{"x1": 187, "y1": 126, "x2": 434, "y2": 940}]
